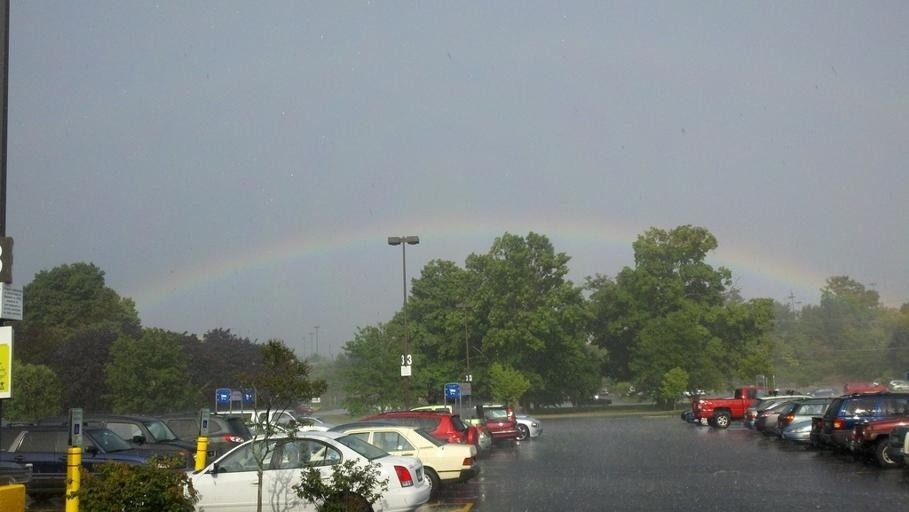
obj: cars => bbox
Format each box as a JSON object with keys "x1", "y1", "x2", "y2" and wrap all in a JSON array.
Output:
[
  {"x1": 680, "y1": 380, "x2": 908, "y2": 475},
  {"x1": 569, "y1": 395, "x2": 613, "y2": 409}
]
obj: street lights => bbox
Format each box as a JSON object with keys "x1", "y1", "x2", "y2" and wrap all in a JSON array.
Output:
[
  {"x1": 301, "y1": 335, "x2": 307, "y2": 359},
  {"x1": 455, "y1": 302, "x2": 476, "y2": 385},
  {"x1": 386, "y1": 236, "x2": 422, "y2": 410},
  {"x1": 313, "y1": 325, "x2": 321, "y2": 353},
  {"x1": 308, "y1": 332, "x2": 314, "y2": 355}
]
